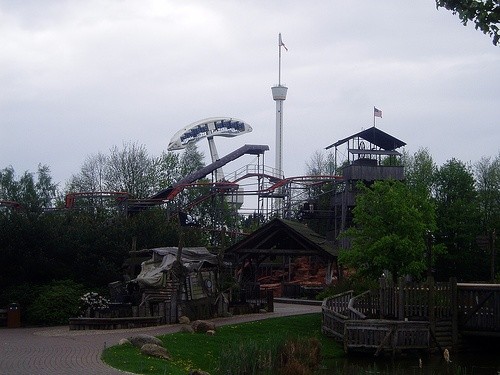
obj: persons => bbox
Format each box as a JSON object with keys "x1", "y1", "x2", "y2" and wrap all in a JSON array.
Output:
[{"x1": 309, "y1": 199, "x2": 315, "y2": 215}]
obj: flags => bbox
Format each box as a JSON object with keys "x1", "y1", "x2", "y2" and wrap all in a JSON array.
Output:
[{"x1": 375, "y1": 107, "x2": 383, "y2": 117}]
